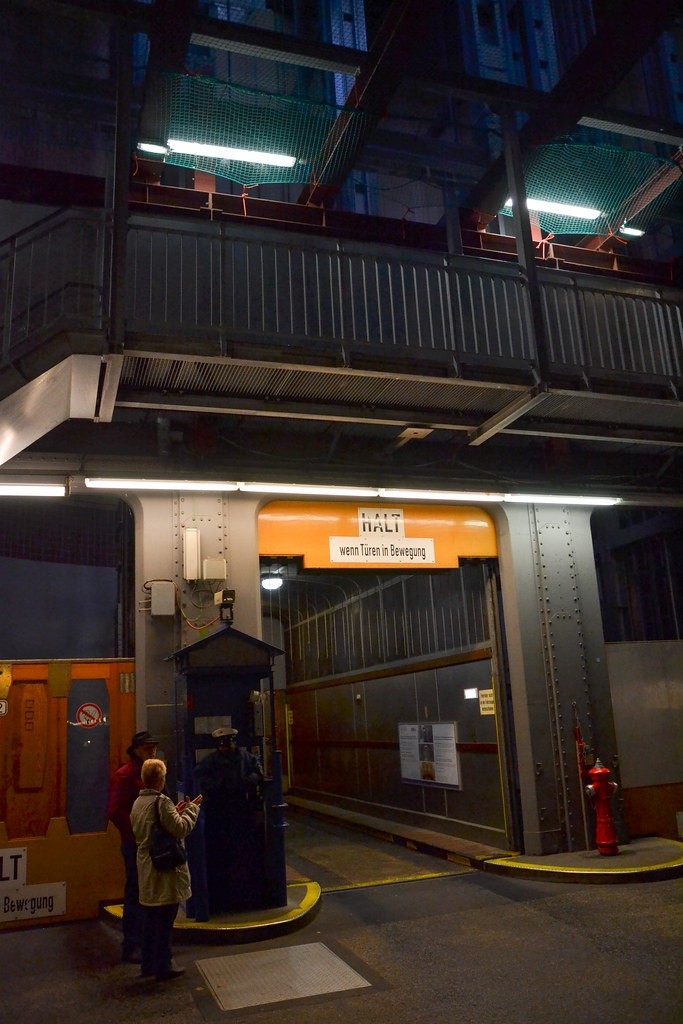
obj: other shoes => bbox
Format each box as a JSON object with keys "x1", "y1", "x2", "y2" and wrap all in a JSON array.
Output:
[
  {"x1": 122, "y1": 951, "x2": 143, "y2": 963},
  {"x1": 155, "y1": 958, "x2": 187, "y2": 977},
  {"x1": 141, "y1": 961, "x2": 156, "y2": 975}
]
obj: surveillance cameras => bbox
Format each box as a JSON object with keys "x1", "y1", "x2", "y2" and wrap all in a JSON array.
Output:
[{"x1": 213, "y1": 588, "x2": 237, "y2": 604}]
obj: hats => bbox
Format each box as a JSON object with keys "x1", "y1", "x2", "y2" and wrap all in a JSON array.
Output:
[
  {"x1": 212, "y1": 727, "x2": 239, "y2": 745},
  {"x1": 127, "y1": 731, "x2": 160, "y2": 755}
]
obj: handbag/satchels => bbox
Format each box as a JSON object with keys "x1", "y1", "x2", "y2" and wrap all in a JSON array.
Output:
[{"x1": 149, "y1": 796, "x2": 187, "y2": 872}]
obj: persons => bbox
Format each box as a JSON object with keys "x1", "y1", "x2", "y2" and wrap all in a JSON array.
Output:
[
  {"x1": 130, "y1": 759, "x2": 202, "y2": 981},
  {"x1": 192, "y1": 728, "x2": 262, "y2": 802},
  {"x1": 109, "y1": 731, "x2": 168, "y2": 964}
]
